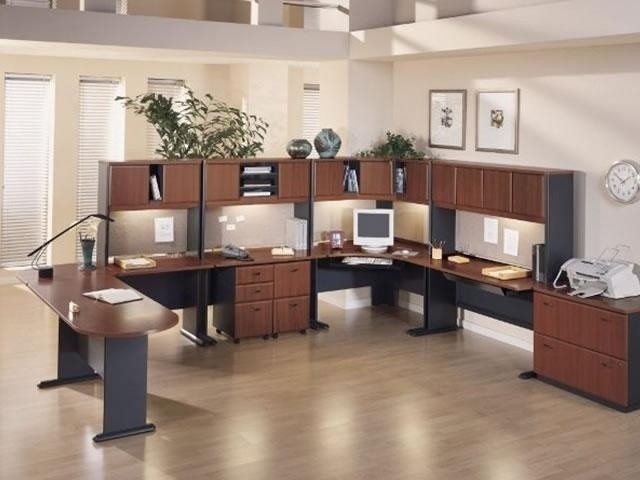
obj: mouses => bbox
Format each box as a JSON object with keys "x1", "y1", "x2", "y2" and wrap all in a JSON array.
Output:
[{"x1": 400, "y1": 249, "x2": 410, "y2": 255}]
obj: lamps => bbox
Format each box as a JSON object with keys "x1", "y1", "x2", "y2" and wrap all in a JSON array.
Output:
[{"x1": 21, "y1": 210, "x2": 115, "y2": 280}]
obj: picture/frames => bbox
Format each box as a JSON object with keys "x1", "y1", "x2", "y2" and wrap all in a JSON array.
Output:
[
  {"x1": 426, "y1": 85, "x2": 468, "y2": 154},
  {"x1": 473, "y1": 87, "x2": 520, "y2": 156}
]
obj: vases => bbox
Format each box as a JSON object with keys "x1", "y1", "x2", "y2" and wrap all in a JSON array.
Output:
[
  {"x1": 313, "y1": 126, "x2": 343, "y2": 159},
  {"x1": 77, "y1": 239, "x2": 97, "y2": 273},
  {"x1": 284, "y1": 138, "x2": 313, "y2": 159}
]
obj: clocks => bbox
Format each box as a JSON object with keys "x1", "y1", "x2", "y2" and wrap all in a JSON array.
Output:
[{"x1": 603, "y1": 158, "x2": 640, "y2": 207}]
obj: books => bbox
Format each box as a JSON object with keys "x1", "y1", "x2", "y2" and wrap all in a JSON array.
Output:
[
  {"x1": 82, "y1": 287, "x2": 144, "y2": 306},
  {"x1": 150, "y1": 174, "x2": 162, "y2": 200},
  {"x1": 271, "y1": 247, "x2": 296, "y2": 257},
  {"x1": 480, "y1": 265, "x2": 533, "y2": 282},
  {"x1": 287, "y1": 217, "x2": 308, "y2": 250},
  {"x1": 115, "y1": 253, "x2": 158, "y2": 272},
  {"x1": 241, "y1": 165, "x2": 273, "y2": 198},
  {"x1": 342, "y1": 165, "x2": 360, "y2": 193}
]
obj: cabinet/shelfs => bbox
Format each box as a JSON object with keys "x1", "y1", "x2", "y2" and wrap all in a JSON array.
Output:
[
  {"x1": 431, "y1": 158, "x2": 576, "y2": 287},
  {"x1": 201, "y1": 158, "x2": 312, "y2": 255},
  {"x1": 312, "y1": 157, "x2": 430, "y2": 258},
  {"x1": 274, "y1": 258, "x2": 314, "y2": 341},
  {"x1": 216, "y1": 259, "x2": 274, "y2": 345},
  {"x1": 531, "y1": 280, "x2": 640, "y2": 409},
  {"x1": 94, "y1": 157, "x2": 201, "y2": 261}
]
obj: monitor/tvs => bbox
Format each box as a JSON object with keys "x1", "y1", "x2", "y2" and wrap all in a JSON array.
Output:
[{"x1": 353, "y1": 208, "x2": 394, "y2": 253}]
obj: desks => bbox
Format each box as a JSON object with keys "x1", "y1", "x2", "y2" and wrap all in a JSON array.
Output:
[
  {"x1": 106, "y1": 250, "x2": 217, "y2": 349},
  {"x1": 309, "y1": 235, "x2": 430, "y2": 338},
  {"x1": 427, "y1": 249, "x2": 536, "y2": 382},
  {"x1": 12, "y1": 259, "x2": 187, "y2": 449},
  {"x1": 205, "y1": 244, "x2": 322, "y2": 346}
]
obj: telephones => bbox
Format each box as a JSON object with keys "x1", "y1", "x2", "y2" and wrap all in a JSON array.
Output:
[
  {"x1": 222, "y1": 244, "x2": 248, "y2": 259},
  {"x1": 561, "y1": 258, "x2": 579, "y2": 271}
]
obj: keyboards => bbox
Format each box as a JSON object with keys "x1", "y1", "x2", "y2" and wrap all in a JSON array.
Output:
[{"x1": 341, "y1": 256, "x2": 393, "y2": 266}]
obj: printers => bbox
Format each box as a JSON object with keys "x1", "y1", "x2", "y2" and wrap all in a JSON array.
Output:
[{"x1": 552, "y1": 247, "x2": 640, "y2": 299}]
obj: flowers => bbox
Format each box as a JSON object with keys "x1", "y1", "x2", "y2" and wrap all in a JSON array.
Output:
[{"x1": 64, "y1": 217, "x2": 106, "y2": 240}]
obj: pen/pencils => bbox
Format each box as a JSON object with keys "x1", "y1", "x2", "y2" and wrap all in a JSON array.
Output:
[{"x1": 429, "y1": 240, "x2": 445, "y2": 248}]
